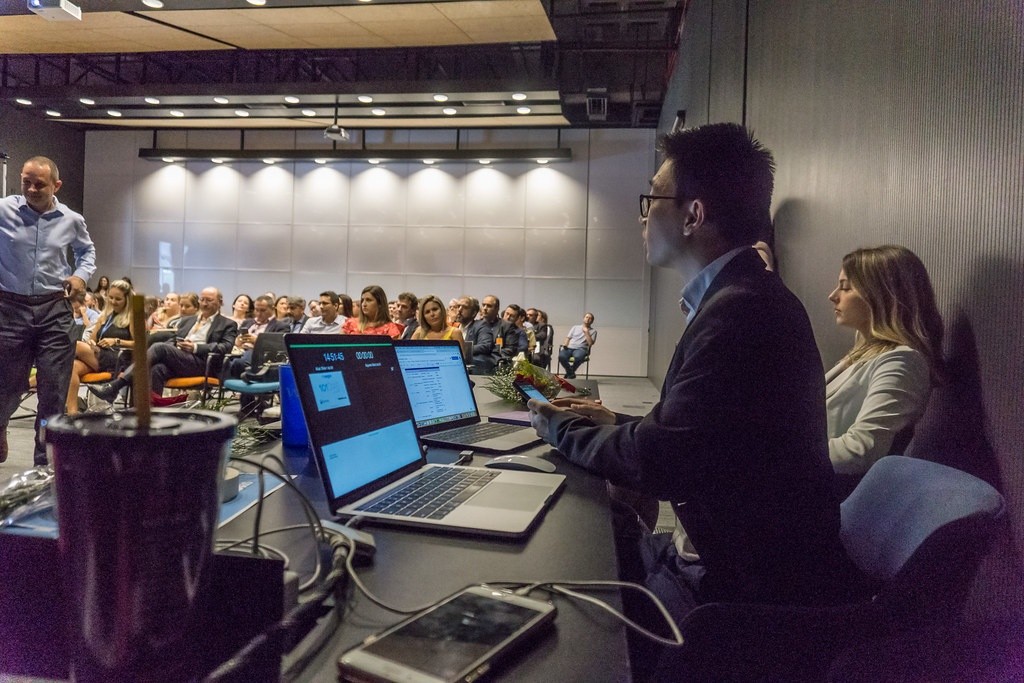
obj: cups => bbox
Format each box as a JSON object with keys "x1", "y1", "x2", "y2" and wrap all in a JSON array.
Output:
[{"x1": 42, "y1": 407, "x2": 238, "y2": 683}]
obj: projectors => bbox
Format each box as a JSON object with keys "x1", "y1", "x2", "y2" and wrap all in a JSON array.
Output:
[
  {"x1": 326, "y1": 127, "x2": 350, "y2": 141},
  {"x1": 27, "y1": 0, "x2": 82, "y2": 22}
]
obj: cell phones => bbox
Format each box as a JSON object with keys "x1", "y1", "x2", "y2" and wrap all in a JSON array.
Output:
[
  {"x1": 241, "y1": 328, "x2": 248, "y2": 336},
  {"x1": 336, "y1": 581, "x2": 558, "y2": 683},
  {"x1": 176, "y1": 337, "x2": 184, "y2": 343},
  {"x1": 512, "y1": 380, "x2": 551, "y2": 405}
]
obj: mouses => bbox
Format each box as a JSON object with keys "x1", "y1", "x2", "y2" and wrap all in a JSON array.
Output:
[{"x1": 485, "y1": 455, "x2": 557, "y2": 473}]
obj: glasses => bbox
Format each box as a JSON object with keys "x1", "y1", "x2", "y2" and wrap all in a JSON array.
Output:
[{"x1": 640, "y1": 193, "x2": 680, "y2": 217}]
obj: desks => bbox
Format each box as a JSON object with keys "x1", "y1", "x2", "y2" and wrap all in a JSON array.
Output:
[{"x1": 0, "y1": 374, "x2": 633, "y2": 683}]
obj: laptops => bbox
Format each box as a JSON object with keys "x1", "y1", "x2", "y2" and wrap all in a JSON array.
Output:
[
  {"x1": 393, "y1": 339, "x2": 545, "y2": 456},
  {"x1": 283, "y1": 332, "x2": 566, "y2": 540}
]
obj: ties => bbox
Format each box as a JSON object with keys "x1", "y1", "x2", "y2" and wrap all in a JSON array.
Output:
[{"x1": 292, "y1": 321, "x2": 299, "y2": 332}]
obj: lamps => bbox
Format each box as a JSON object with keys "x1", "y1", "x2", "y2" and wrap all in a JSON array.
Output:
[{"x1": 323, "y1": 106, "x2": 350, "y2": 142}]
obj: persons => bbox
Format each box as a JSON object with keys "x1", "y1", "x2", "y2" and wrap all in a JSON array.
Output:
[
  {"x1": 66, "y1": 275, "x2": 137, "y2": 414},
  {"x1": 824, "y1": 245, "x2": 945, "y2": 474},
  {"x1": 341, "y1": 285, "x2": 466, "y2": 358},
  {"x1": 528, "y1": 125, "x2": 843, "y2": 662},
  {"x1": 515, "y1": 307, "x2": 548, "y2": 364},
  {"x1": 447, "y1": 295, "x2": 519, "y2": 373},
  {"x1": 86, "y1": 285, "x2": 238, "y2": 403},
  {"x1": 219, "y1": 291, "x2": 353, "y2": 418},
  {"x1": 0, "y1": 157, "x2": 97, "y2": 468},
  {"x1": 558, "y1": 313, "x2": 598, "y2": 379}
]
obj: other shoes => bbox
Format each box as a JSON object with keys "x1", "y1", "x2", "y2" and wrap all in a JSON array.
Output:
[
  {"x1": 0, "y1": 430, "x2": 10, "y2": 461},
  {"x1": 34, "y1": 437, "x2": 49, "y2": 464}
]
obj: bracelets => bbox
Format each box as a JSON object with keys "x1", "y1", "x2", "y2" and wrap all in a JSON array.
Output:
[{"x1": 115, "y1": 338, "x2": 120, "y2": 346}]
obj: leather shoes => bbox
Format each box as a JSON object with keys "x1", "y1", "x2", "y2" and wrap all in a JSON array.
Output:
[{"x1": 87, "y1": 383, "x2": 120, "y2": 403}]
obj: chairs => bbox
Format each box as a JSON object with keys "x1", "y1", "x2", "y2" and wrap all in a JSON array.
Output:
[
  {"x1": 8, "y1": 326, "x2": 589, "y2": 419},
  {"x1": 645, "y1": 426, "x2": 1007, "y2": 682}
]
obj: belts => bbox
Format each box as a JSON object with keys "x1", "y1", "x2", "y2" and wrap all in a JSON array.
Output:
[{"x1": 0, "y1": 291, "x2": 65, "y2": 305}]
